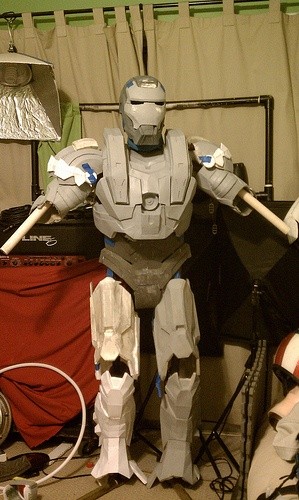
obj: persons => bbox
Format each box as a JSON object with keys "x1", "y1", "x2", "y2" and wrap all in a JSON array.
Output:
[{"x1": 1, "y1": 75, "x2": 289, "y2": 487}]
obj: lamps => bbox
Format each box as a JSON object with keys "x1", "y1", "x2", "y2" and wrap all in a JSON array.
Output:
[{"x1": 1, "y1": 46, "x2": 64, "y2": 143}]
